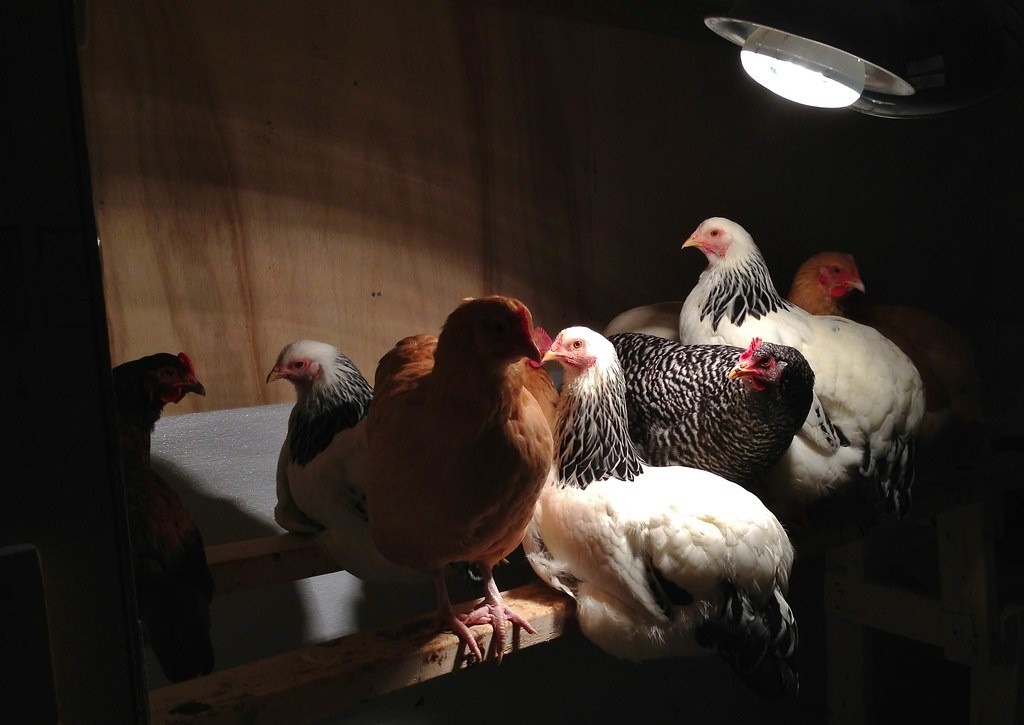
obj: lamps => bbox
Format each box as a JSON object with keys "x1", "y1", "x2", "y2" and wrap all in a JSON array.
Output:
[{"x1": 702, "y1": 5, "x2": 916, "y2": 111}]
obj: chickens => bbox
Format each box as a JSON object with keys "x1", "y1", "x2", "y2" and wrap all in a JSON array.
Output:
[
  {"x1": 113, "y1": 353, "x2": 217, "y2": 686},
  {"x1": 522, "y1": 328, "x2": 804, "y2": 698},
  {"x1": 788, "y1": 251, "x2": 866, "y2": 318},
  {"x1": 363, "y1": 294, "x2": 555, "y2": 667},
  {"x1": 680, "y1": 215, "x2": 925, "y2": 522},
  {"x1": 264, "y1": 337, "x2": 375, "y2": 534},
  {"x1": 371, "y1": 323, "x2": 561, "y2": 440},
  {"x1": 605, "y1": 333, "x2": 815, "y2": 488}
]
